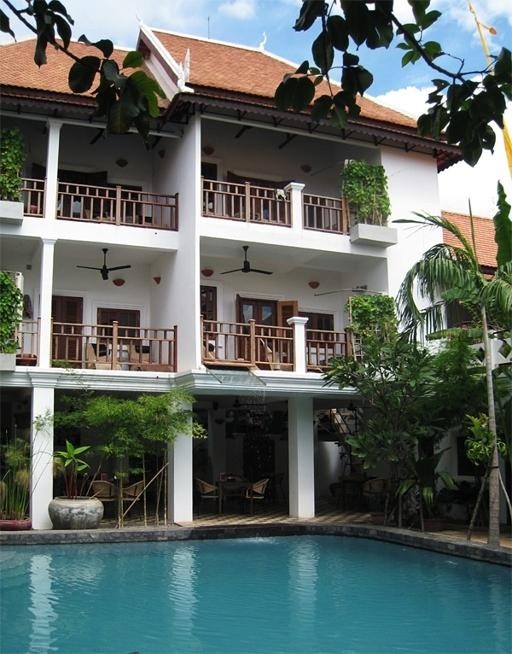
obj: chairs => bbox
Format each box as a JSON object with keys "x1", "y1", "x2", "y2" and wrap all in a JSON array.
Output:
[
  {"x1": 85, "y1": 343, "x2": 121, "y2": 370},
  {"x1": 201, "y1": 339, "x2": 257, "y2": 370},
  {"x1": 127, "y1": 344, "x2": 171, "y2": 372},
  {"x1": 195, "y1": 472, "x2": 270, "y2": 516},
  {"x1": 89, "y1": 472, "x2": 146, "y2": 521},
  {"x1": 259, "y1": 338, "x2": 323, "y2": 373}
]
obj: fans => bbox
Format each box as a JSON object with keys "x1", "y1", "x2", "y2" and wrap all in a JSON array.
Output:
[
  {"x1": 74, "y1": 248, "x2": 132, "y2": 281},
  {"x1": 219, "y1": 245, "x2": 274, "y2": 276}
]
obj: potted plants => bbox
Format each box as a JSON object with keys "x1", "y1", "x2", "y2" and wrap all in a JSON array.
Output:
[
  {"x1": 49, "y1": 440, "x2": 104, "y2": 531},
  {"x1": 0, "y1": 409, "x2": 53, "y2": 532}
]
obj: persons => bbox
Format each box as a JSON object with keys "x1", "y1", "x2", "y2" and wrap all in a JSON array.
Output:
[{"x1": 100, "y1": 472, "x2": 114, "y2": 482}]
